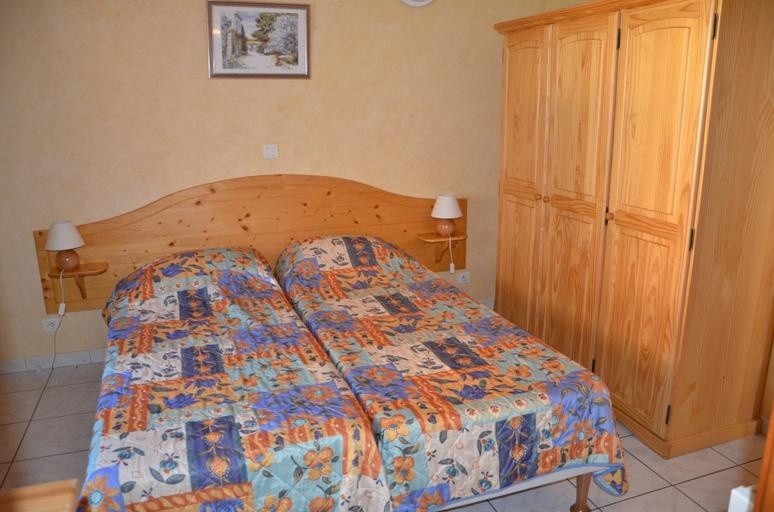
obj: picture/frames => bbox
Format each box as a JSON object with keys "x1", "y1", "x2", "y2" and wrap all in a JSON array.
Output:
[{"x1": 206, "y1": 1, "x2": 311, "y2": 79}]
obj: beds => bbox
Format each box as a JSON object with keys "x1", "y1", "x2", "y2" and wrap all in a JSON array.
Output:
[{"x1": 75, "y1": 235, "x2": 615, "y2": 512}]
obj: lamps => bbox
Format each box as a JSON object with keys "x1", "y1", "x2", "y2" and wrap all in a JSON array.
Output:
[
  {"x1": 431, "y1": 193, "x2": 464, "y2": 238},
  {"x1": 45, "y1": 218, "x2": 87, "y2": 274}
]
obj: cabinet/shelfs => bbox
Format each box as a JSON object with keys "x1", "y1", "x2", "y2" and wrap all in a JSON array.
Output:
[{"x1": 490, "y1": 1, "x2": 774, "y2": 462}]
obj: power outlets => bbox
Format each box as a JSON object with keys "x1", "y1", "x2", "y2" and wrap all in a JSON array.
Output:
[
  {"x1": 42, "y1": 317, "x2": 62, "y2": 334},
  {"x1": 458, "y1": 272, "x2": 471, "y2": 284}
]
